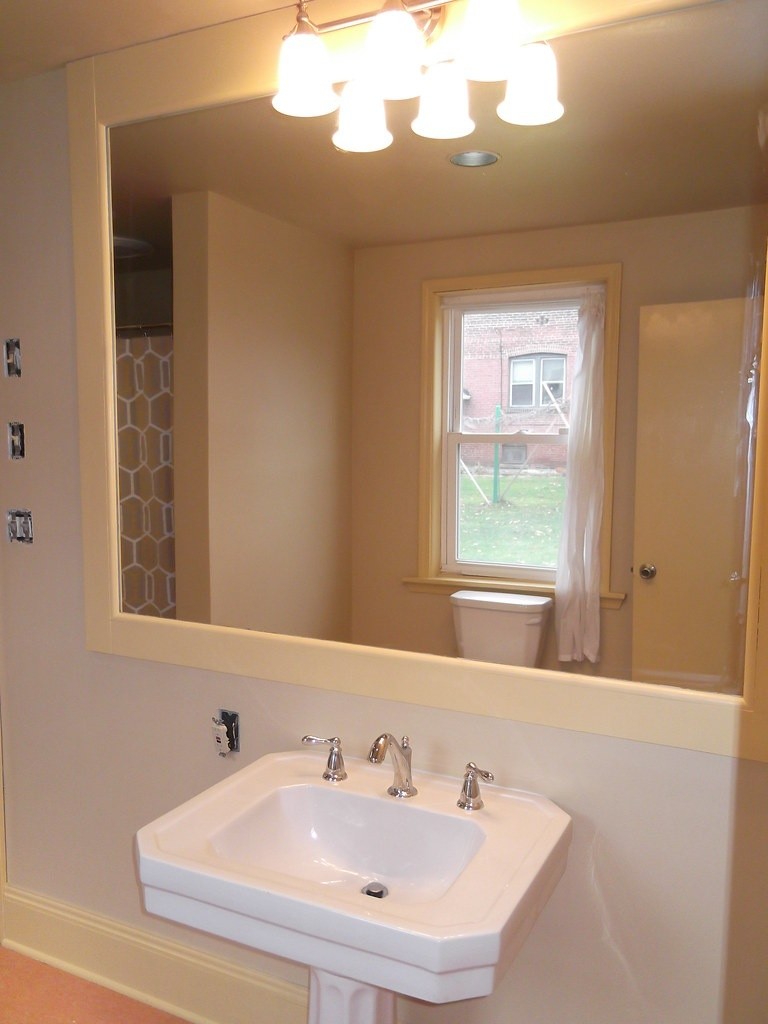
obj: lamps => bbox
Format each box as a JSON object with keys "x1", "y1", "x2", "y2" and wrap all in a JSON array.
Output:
[
  {"x1": 332, "y1": 40, "x2": 565, "y2": 153},
  {"x1": 270, "y1": 0, "x2": 527, "y2": 117}
]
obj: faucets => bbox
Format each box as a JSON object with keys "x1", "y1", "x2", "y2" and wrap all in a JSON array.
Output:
[{"x1": 368, "y1": 731, "x2": 418, "y2": 798}]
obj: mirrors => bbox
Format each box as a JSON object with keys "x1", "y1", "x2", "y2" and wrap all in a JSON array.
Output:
[{"x1": 64, "y1": 0, "x2": 768, "y2": 767}]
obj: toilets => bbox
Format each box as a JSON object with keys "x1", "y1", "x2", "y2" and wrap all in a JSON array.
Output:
[{"x1": 448, "y1": 590, "x2": 553, "y2": 668}]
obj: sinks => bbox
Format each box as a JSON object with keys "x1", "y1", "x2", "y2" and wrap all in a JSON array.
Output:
[{"x1": 134, "y1": 751, "x2": 574, "y2": 1004}]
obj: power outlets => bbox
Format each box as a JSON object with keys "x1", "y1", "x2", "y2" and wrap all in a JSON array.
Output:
[{"x1": 211, "y1": 708, "x2": 241, "y2": 760}]
